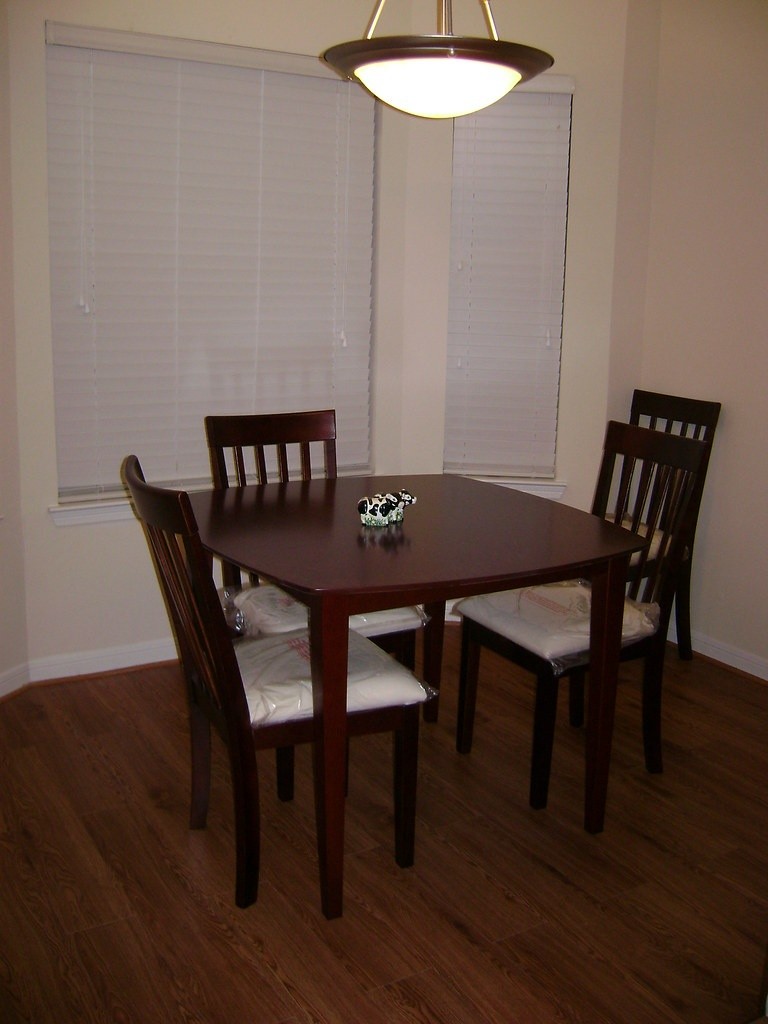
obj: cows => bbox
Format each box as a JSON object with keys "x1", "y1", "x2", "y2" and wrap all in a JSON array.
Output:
[{"x1": 358, "y1": 488, "x2": 418, "y2": 525}]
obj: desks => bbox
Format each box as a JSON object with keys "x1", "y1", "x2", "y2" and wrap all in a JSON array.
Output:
[{"x1": 189, "y1": 474, "x2": 650, "y2": 920}]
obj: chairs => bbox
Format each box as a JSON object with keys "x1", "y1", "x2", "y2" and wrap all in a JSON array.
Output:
[
  {"x1": 456, "y1": 420, "x2": 710, "y2": 810},
  {"x1": 120, "y1": 455, "x2": 427, "y2": 910},
  {"x1": 605, "y1": 388, "x2": 722, "y2": 663},
  {"x1": 204, "y1": 410, "x2": 422, "y2": 798}
]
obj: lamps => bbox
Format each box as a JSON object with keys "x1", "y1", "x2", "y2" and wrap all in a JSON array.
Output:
[{"x1": 322, "y1": 0, "x2": 553, "y2": 119}]
